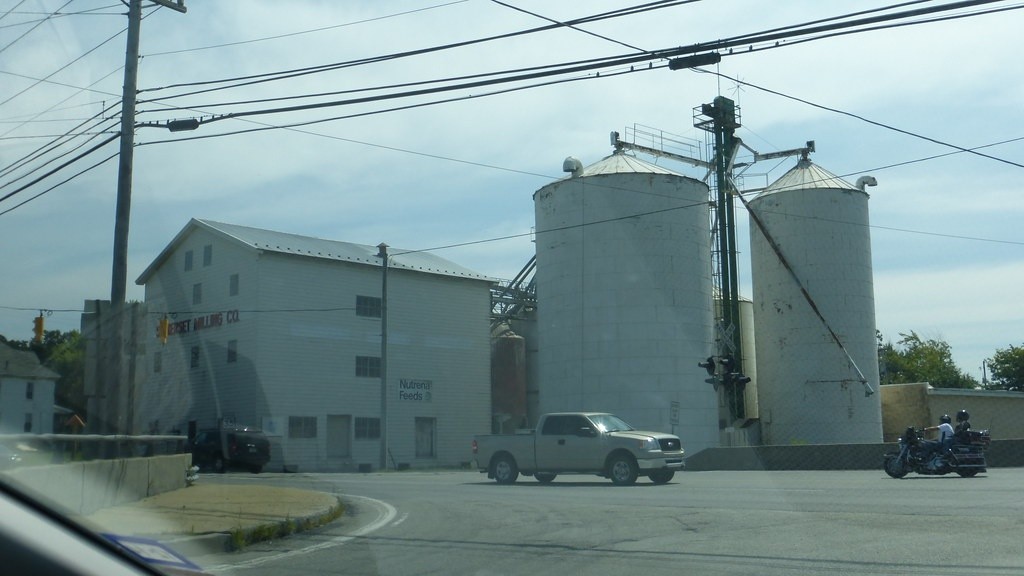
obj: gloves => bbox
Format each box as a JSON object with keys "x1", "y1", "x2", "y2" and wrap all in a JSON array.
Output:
[{"x1": 916, "y1": 428, "x2": 923, "y2": 432}]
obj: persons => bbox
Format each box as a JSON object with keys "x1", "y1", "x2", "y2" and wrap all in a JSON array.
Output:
[{"x1": 916, "y1": 409, "x2": 970, "y2": 462}]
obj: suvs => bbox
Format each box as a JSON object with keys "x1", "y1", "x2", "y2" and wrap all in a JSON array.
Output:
[{"x1": 184, "y1": 422, "x2": 271, "y2": 474}]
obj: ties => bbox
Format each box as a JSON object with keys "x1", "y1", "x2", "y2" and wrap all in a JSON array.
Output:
[{"x1": 942, "y1": 431, "x2": 945, "y2": 444}]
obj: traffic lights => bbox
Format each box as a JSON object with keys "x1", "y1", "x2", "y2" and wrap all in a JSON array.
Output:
[{"x1": 31, "y1": 315, "x2": 45, "y2": 342}]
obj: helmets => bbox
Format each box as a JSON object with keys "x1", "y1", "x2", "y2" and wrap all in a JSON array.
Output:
[
  {"x1": 939, "y1": 414, "x2": 951, "y2": 424},
  {"x1": 956, "y1": 411, "x2": 969, "y2": 420}
]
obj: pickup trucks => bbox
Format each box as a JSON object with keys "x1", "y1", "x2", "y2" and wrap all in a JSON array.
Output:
[{"x1": 472, "y1": 412, "x2": 685, "y2": 486}]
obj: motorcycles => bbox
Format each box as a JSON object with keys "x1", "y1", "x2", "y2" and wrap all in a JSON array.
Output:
[{"x1": 882, "y1": 426, "x2": 991, "y2": 479}]
